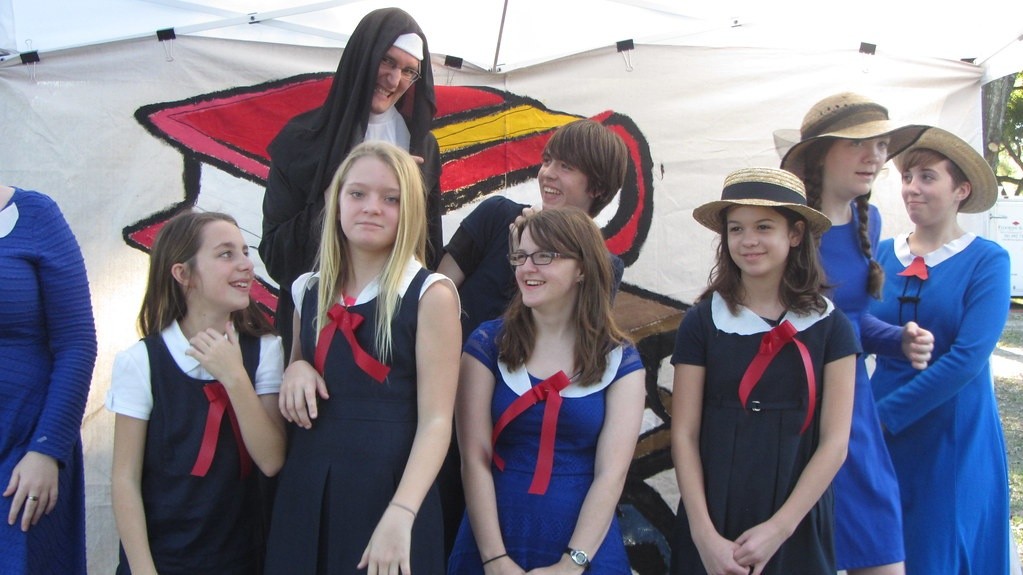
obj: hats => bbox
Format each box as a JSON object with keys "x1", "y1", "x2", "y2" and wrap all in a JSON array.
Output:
[
  {"x1": 780, "y1": 91, "x2": 934, "y2": 187},
  {"x1": 891, "y1": 125, "x2": 999, "y2": 213},
  {"x1": 692, "y1": 170, "x2": 832, "y2": 238}
]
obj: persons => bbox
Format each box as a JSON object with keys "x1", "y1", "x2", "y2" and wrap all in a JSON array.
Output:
[
  {"x1": 269, "y1": 140, "x2": 464, "y2": 575},
  {"x1": 0, "y1": 186, "x2": 99, "y2": 575},
  {"x1": 439, "y1": 120, "x2": 627, "y2": 346},
  {"x1": 781, "y1": 92, "x2": 936, "y2": 574},
  {"x1": 443, "y1": 204, "x2": 649, "y2": 575},
  {"x1": 866, "y1": 124, "x2": 1020, "y2": 574},
  {"x1": 258, "y1": 6, "x2": 442, "y2": 365},
  {"x1": 104, "y1": 211, "x2": 285, "y2": 574},
  {"x1": 663, "y1": 168, "x2": 862, "y2": 574}
]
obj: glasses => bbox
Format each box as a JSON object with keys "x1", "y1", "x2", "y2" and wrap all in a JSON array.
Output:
[
  {"x1": 506, "y1": 247, "x2": 573, "y2": 266},
  {"x1": 380, "y1": 54, "x2": 423, "y2": 83}
]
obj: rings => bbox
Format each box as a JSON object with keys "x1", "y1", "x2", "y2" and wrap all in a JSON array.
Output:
[{"x1": 28, "y1": 496, "x2": 39, "y2": 501}]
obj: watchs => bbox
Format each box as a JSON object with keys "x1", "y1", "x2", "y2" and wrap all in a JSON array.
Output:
[{"x1": 563, "y1": 548, "x2": 590, "y2": 570}]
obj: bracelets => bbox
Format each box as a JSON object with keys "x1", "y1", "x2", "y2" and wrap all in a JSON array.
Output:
[
  {"x1": 482, "y1": 553, "x2": 507, "y2": 566},
  {"x1": 390, "y1": 502, "x2": 416, "y2": 517}
]
